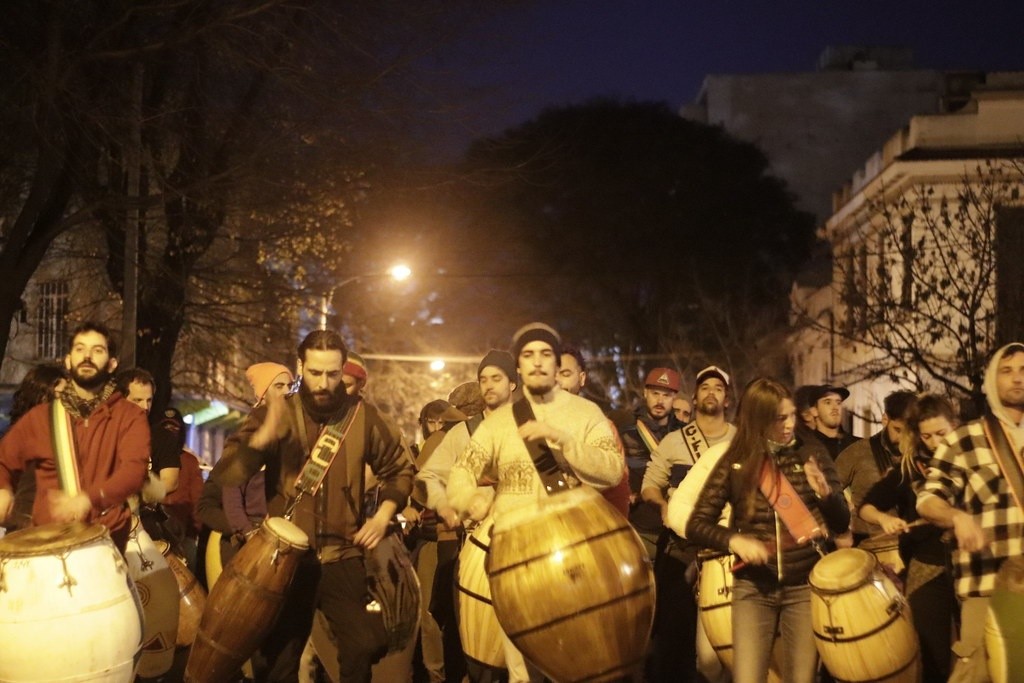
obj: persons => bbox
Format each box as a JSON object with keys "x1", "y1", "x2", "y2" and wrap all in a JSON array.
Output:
[{"x1": 0, "y1": 322, "x2": 1024, "y2": 683}]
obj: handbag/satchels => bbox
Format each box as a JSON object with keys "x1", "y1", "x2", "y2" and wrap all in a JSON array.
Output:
[{"x1": 363, "y1": 517, "x2": 422, "y2": 659}]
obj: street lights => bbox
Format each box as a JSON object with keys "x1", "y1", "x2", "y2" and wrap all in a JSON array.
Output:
[{"x1": 320, "y1": 263, "x2": 412, "y2": 329}]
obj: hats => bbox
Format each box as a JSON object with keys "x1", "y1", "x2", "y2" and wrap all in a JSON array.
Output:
[
  {"x1": 449, "y1": 382, "x2": 486, "y2": 416},
  {"x1": 341, "y1": 349, "x2": 368, "y2": 389},
  {"x1": 810, "y1": 384, "x2": 850, "y2": 408},
  {"x1": 646, "y1": 367, "x2": 681, "y2": 393},
  {"x1": 245, "y1": 363, "x2": 294, "y2": 401},
  {"x1": 478, "y1": 350, "x2": 519, "y2": 392},
  {"x1": 159, "y1": 408, "x2": 183, "y2": 428},
  {"x1": 696, "y1": 366, "x2": 729, "y2": 387},
  {"x1": 511, "y1": 321, "x2": 562, "y2": 368}
]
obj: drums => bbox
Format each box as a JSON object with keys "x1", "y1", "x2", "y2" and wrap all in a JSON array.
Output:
[
  {"x1": 485, "y1": 489, "x2": 657, "y2": 683},
  {"x1": 124, "y1": 512, "x2": 180, "y2": 678},
  {"x1": 983, "y1": 555, "x2": 1024, "y2": 683},
  {"x1": 183, "y1": 517, "x2": 310, "y2": 683},
  {"x1": 808, "y1": 548, "x2": 923, "y2": 683},
  {"x1": 627, "y1": 492, "x2": 659, "y2": 562},
  {"x1": 153, "y1": 539, "x2": 207, "y2": 648},
  {"x1": 698, "y1": 546, "x2": 736, "y2": 672},
  {"x1": 205, "y1": 530, "x2": 254, "y2": 679},
  {"x1": 861, "y1": 526, "x2": 904, "y2": 574},
  {"x1": 456, "y1": 509, "x2": 506, "y2": 671},
  {"x1": 695, "y1": 608, "x2": 732, "y2": 683},
  {"x1": 308, "y1": 524, "x2": 423, "y2": 683},
  {"x1": 0, "y1": 523, "x2": 147, "y2": 683}
]
